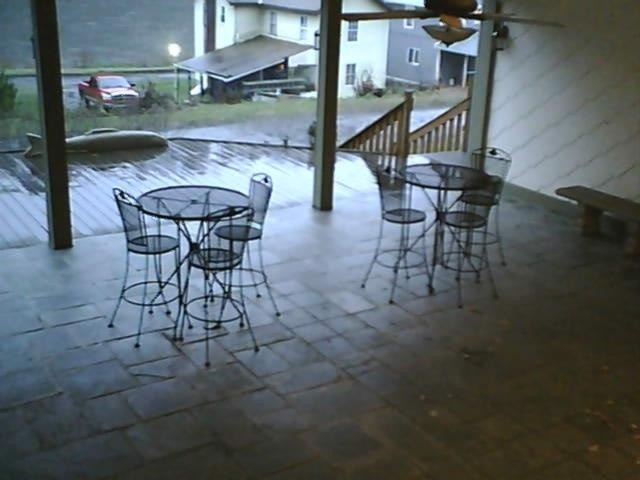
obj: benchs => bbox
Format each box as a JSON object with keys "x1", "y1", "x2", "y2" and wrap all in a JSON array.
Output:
[{"x1": 555, "y1": 185, "x2": 640, "y2": 236}]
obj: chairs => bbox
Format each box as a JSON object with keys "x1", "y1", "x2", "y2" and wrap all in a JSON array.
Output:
[
  {"x1": 107, "y1": 173, "x2": 281, "y2": 368},
  {"x1": 362, "y1": 146, "x2": 512, "y2": 307}
]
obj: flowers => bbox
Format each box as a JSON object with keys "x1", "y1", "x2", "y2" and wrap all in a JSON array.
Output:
[{"x1": 492, "y1": 24, "x2": 509, "y2": 38}]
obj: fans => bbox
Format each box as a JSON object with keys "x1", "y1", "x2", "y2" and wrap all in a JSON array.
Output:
[{"x1": 342, "y1": 0, "x2": 570, "y2": 27}]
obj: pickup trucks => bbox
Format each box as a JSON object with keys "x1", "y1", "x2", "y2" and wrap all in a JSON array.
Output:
[{"x1": 72, "y1": 66, "x2": 154, "y2": 122}]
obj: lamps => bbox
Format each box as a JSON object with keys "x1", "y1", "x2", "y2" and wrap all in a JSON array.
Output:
[{"x1": 423, "y1": 17, "x2": 476, "y2": 44}]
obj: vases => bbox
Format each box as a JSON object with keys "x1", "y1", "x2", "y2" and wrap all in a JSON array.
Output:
[{"x1": 495, "y1": 38, "x2": 504, "y2": 49}]
